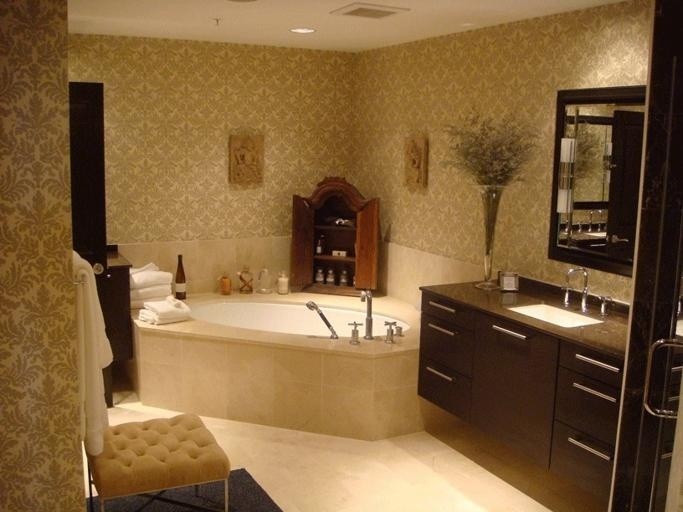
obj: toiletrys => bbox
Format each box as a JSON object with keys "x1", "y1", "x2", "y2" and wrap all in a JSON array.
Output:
[{"x1": 275, "y1": 269, "x2": 289, "y2": 295}]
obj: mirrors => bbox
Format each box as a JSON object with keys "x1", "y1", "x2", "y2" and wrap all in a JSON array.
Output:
[{"x1": 545, "y1": 83, "x2": 647, "y2": 279}]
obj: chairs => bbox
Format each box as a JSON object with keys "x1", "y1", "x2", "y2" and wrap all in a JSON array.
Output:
[{"x1": 83, "y1": 411, "x2": 230, "y2": 512}]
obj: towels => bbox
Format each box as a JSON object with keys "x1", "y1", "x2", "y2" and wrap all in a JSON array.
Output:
[
  {"x1": 138, "y1": 296, "x2": 190, "y2": 326},
  {"x1": 130, "y1": 262, "x2": 172, "y2": 310}
]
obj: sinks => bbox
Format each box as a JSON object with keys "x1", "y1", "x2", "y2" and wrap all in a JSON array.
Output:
[
  {"x1": 505, "y1": 302, "x2": 606, "y2": 328},
  {"x1": 583, "y1": 232, "x2": 606, "y2": 237}
]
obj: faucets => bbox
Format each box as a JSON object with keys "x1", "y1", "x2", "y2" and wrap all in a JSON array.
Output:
[
  {"x1": 559, "y1": 266, "x2": 590, "y2": 313},
  {"x1": 360, "y1": 289, "x2": 375, "y2": 341},
  {"x1": 588, "y1": 209, "x2": 603, "y2": 232}
]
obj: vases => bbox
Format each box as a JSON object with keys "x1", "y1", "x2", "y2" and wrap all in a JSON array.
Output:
[{"x1": 474, "y1": 188, "x2": 505, "y2": 291}]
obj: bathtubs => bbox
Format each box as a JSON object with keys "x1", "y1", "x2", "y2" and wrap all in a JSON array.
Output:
[{"x1": 129, "y1": 290, "x2": 419, "y2": 444}]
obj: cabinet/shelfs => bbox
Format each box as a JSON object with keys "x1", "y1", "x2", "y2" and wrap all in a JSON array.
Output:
[
  {"x1": 68, "y1": 82, "x2": 134, "y2": 411},
  {"x1": 415, "y1": 278, "x2": 629, "y2": 498},
  {"x1": 289, "y1": 175, "x2": 380, "y2": 297}
]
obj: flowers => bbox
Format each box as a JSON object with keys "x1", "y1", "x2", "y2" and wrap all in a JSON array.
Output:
[{"x1": 445, "y1": 109, "x2": 538, "y2": 186}]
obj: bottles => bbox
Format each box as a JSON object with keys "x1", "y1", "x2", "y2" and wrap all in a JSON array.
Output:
[
  {"x1": 326, "y1": 270, "x2": 335, "y2": 284},
  {"x1": 278, "y1": 271, "x2": 289, "y2": 294},
  {"x1": 221, "y1": 273, "x2": 230, "y2": 295},
  {"x1": 339, "y1": 271, "x2": 348, "y2": 285},
  {"x1": 315, "y1": 237, "x2": 323, "y2": 255},
  {"x1": 240, "y1": 267, "x2": 253, "y2": 293},
  {"x1": 175, "y1": 255, "x2": 186, "y2": 300},
  {"x1": 258, "y1": 268, "x2": 272, "y2": 294},
  {"x1": 315, "y1": 270, "x2": 324, "y2": 283}
]
